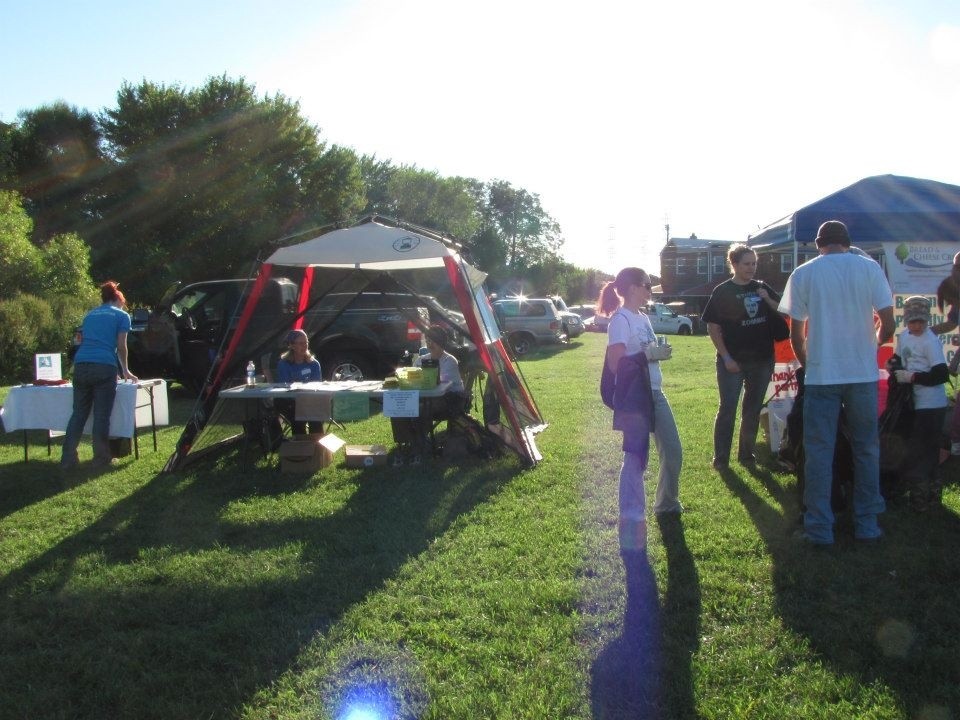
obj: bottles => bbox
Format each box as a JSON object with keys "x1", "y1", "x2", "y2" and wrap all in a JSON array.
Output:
[{"x1": 246, "y1": 361, "x2": 256, "y2": 385}]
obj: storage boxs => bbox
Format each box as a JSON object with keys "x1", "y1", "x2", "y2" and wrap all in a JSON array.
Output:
[
  {"x1": 346, "y1": 445, "x2": 389, "y2": 468},
  {"x1": 279, "y1": 434, "x2": 346, "y2": 475},
  {"x1": 396, "y1": 367, "x2": 439, "y2": 388}
]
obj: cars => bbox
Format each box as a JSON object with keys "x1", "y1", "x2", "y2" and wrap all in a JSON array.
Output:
[
  {"x1": 462, "y1": 298, "x2": 568, "y2": 355},
  {"x1": 547, "y1": 296, "x2": 609, "y2": 339},
  {"x1": 315, "y1": 289, "x2": 477, "y2": 368}
]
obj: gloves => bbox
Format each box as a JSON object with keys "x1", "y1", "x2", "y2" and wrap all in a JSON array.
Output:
[{"x1": 895, "y1": 370, "x2": 914, "y2": 384}]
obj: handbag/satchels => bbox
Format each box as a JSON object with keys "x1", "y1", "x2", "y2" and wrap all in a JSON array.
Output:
[{"x1": 768, "y1": 309, "x2": 791, "y2": 342}]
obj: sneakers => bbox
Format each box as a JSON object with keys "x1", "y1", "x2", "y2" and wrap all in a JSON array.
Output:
[
  {"x1": 654, "y1": 502, "x2": 684, "y2": 513},
  {"x1": 619, "y1": 511, "x2": 647, "y2": 522}
]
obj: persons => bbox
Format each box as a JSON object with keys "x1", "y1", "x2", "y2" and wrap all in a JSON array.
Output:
[
  {"x1": 597, "y1": 266, "x2": 683, "y2": 554},
  {"x1": 403, "y1": 325, "x2": 464, "y2": 437},
  {"x1": 886, "y1": 296, "x2": 951, "y2": 507},
  {"x1": 927, "y1": 252, "x2": 959, "y2": 473},
  {"x1": 61, "y1": 280, "x2": 139, "y2": 473},
  {"x1": 700, "y1": 242, "x2": 792, "y2": 470},
  {"x1": 273, "y1": 328, "x2": 326, "y2": 436},
  {"x1": 777, "y1": 219, "x2": 897, "y2": 542}
]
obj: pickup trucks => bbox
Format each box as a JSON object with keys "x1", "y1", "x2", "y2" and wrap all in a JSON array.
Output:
[
  {"x1": 645, "y1": 303, "x2": 694, "y2": 336},
  {"x1": 119, "y1": 279, "x2": 430, "y2": 392}
]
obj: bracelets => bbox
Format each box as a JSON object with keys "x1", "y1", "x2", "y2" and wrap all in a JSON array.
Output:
[{"x1": 722, "y1": 353, "x2": 732, "y2": 363}]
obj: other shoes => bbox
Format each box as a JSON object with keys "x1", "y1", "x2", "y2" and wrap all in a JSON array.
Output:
[
  {"x1": 855, "y1": 528, "x2": 887, "y2": 543},
  {"x1": 800, "y1": 530, "x2": 836, "y2": 548},
  {"x1": 912, "y1": 493, "x2": 942, "y2": 513},
  {"x1": 714, "y1": 456, "x2": 729, "y2": 470},
  {"x1": 740, "y1": 454, "x2": 755, "y2": 462}
]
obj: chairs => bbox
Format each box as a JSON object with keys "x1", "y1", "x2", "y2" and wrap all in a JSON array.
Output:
[
  {"x1": 397, "y1": 360, "x2": 486, "y2": 454},
  {"x1": 264, "y1": 372, "x2": 299, "y2": 451}
]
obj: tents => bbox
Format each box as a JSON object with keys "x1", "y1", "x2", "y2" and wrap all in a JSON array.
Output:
[
  {"x1": 748, "y1": 174, "x2": 960, "y2": 296},
  {"x1": 158, "y1": 212, "x2": 550, "y2": 479}
]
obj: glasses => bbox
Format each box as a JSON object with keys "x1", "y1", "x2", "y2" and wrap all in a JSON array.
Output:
[{"x1": 640, "y1": 283, "x2": 652, "y2": 290}]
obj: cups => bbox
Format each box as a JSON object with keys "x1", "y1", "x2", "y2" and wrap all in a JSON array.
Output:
[{"x1": 657, "y1": 336, "x2": 668, "y2": 347}]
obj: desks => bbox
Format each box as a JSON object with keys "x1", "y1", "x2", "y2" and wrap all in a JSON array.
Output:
[
  {"x1": 218, "y1": 381, "x2": 461, "y2": 479},
  {"x1": 761, "y1": 362, "x2": 890, "y2": 452},
  {"x1": 0, "y1": 378, "x2": 169, "y2": 462}
]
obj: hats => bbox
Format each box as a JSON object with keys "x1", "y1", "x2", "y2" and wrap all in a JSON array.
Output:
[
  {"x1": 816, "y1": 221, "x2": 851, "y2": 248},
  {"x1": 904, "y1": 295, "x2": 932, "y2": 327}
]
